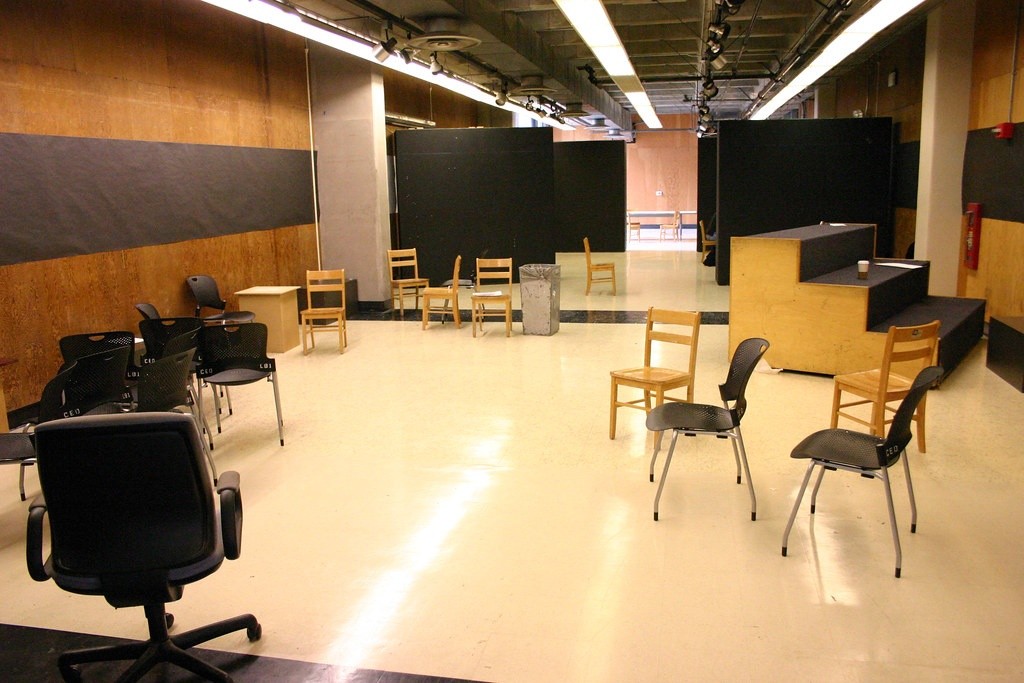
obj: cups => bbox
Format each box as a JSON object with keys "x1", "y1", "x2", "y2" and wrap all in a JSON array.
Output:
[{"x1": 858, "y1": 260, "x2": 869, "y2": 279}]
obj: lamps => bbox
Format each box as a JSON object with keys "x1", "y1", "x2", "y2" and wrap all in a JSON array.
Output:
[
  {"x1": 373, "y1": 38, "x2": 398, "y2": 63},
  {"x1": 526, "y1": 96, "x2": 566, "y2": 124},
  {"x1": 430, "y1": 51, "x2": 444, "y2": 76},
  {"x1": 694, "y1": 0, "x2": 745, "y2": 139},
  {"x1": 399, "y1": 46, "x2": 422, "y2": 64},
  {"x1": 495, "y1": 89, "x2": 506, "y2": 106}
]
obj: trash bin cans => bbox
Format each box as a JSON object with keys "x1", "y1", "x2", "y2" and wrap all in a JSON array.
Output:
[{"x1": 518, "y1": 264, "x2": 561, "y2": 335}]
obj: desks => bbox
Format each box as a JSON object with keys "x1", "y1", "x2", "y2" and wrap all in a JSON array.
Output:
[{"x1": 233, "y1": 286, "x2": 301, "y2": 354}]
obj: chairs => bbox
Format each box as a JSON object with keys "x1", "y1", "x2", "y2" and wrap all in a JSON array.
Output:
[
  {"x1": 471, "y1": 258, "x2": 513, "y2": 338},
  {"x1": 699, "y1": 220, "x2": 717, "y2": 263},
  {"x1": 300, "y1": 268, "x2": 348, "y2": 356},
  {"x1": 829, "y1": 319, "x2": 941, "y2": 453},
  {"x1": 386, "y1": 248, "x2": 430, "y2": 316},
  {"x1": 422, "y1": 254, "x2": 462, "y2": 331},
  {"x1": 782, "y1": 366, "x2": 944, "y2": 578},
  {"x1": 0, "y1": 274, "x2": 284, "y2": 683},
  {"x1": 583, "y1": 237, "x2": 616, "y2": 296},
  {"x1": 609, "y1": 307, "x2": 703, "y2": 451},
  {"x1": 628, "y1": 213, "x2": 640, "y2": 238},
  {"x1": 646, "y1": 338, "x2": 770, "y2": 521},
  {"x1": 660, "y1": 210, "x2": 680, "y2": 243}
]
